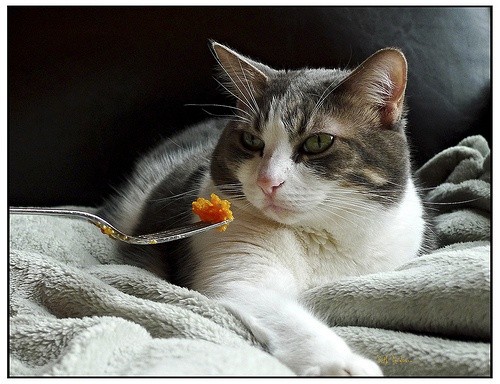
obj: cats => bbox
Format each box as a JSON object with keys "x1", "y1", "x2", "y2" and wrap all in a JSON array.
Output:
[{"x1": 92, "y1": 39, "x2": 484, "y2": 377}]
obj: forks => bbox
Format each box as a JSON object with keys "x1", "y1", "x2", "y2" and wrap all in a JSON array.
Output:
[{"x1": 8, "y1": 208, "x2": 233, "y2": 246}]
